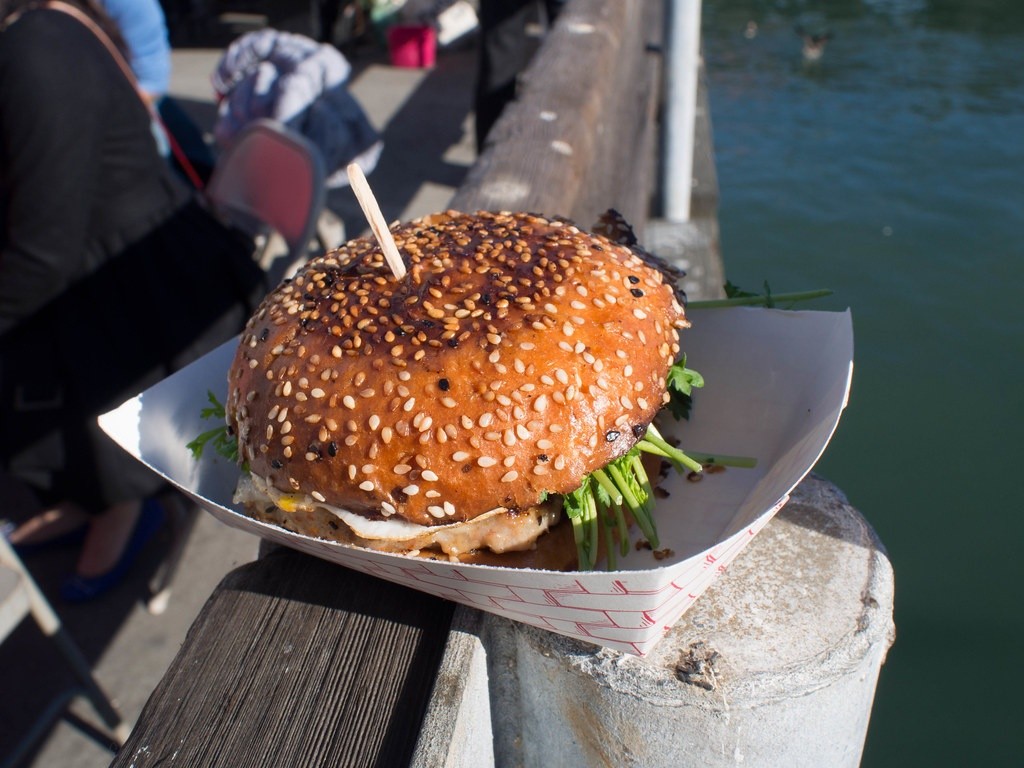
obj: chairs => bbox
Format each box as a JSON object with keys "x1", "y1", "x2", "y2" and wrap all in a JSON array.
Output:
[{"x1": 0, "y1": 121, "x2": 328, "y2": 768}]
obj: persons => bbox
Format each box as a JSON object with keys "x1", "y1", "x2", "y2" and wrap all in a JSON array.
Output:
[
  {"x1": 0, "y1": 0, "x2": 267, "y2": 608},
  {"x1": 103, "y1": 0, "x2": 171, "y2": 100},
  {"x1": 473, "y1": 0, "x2": 565, "y2": 155}
]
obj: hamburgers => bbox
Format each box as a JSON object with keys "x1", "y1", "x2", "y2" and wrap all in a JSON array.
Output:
[{"x1": 182, "y1": 211, "x2": 837, "y2": 570}]
